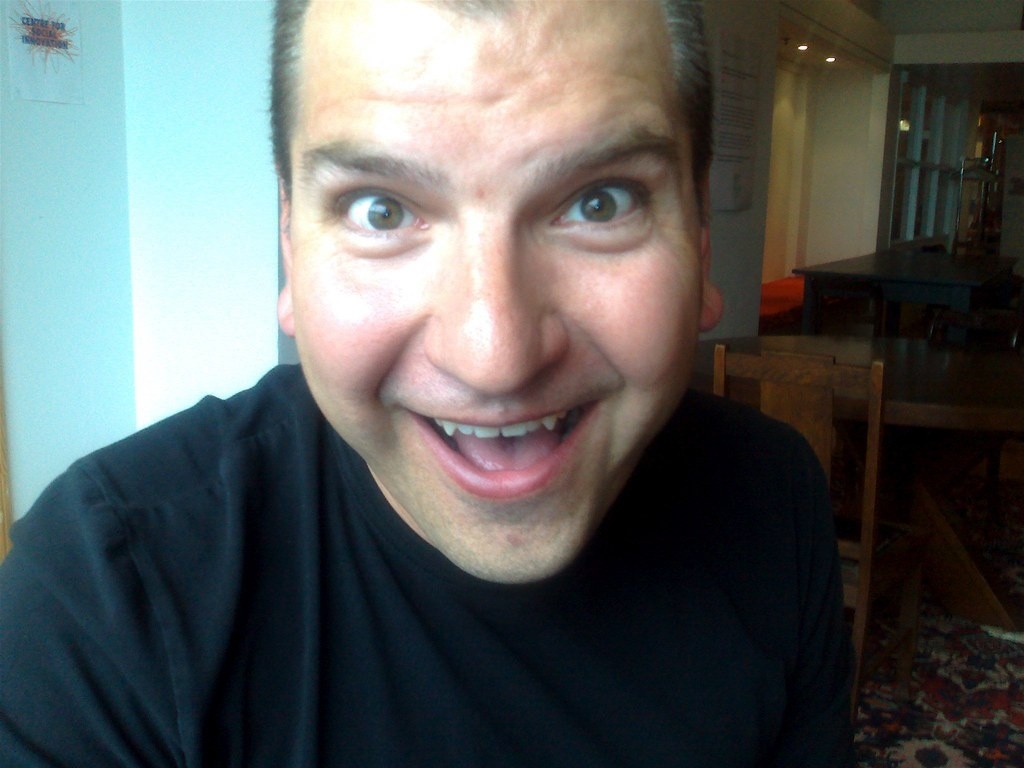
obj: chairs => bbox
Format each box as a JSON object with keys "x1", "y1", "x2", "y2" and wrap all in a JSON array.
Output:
[{"x1": 713, "y1": 343, "x2": 932, "y2": 724}]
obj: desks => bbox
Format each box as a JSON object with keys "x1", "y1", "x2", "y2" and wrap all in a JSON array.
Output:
[
  {"x1": 791, "y1": 251, "x2": 1019, "y2": 340},
  {"x1": 690, "y1": 335, "x2": 1024, "y2": 632}
]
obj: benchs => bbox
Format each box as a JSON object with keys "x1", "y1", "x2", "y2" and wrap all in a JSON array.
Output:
[{"x1": 758, "y1": 276, "x2": 857, "y2": 335}]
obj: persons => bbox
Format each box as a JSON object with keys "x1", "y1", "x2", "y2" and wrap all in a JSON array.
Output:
[{"x1": 0, "y1": 0, "x2": 850, "y2": 767}]
